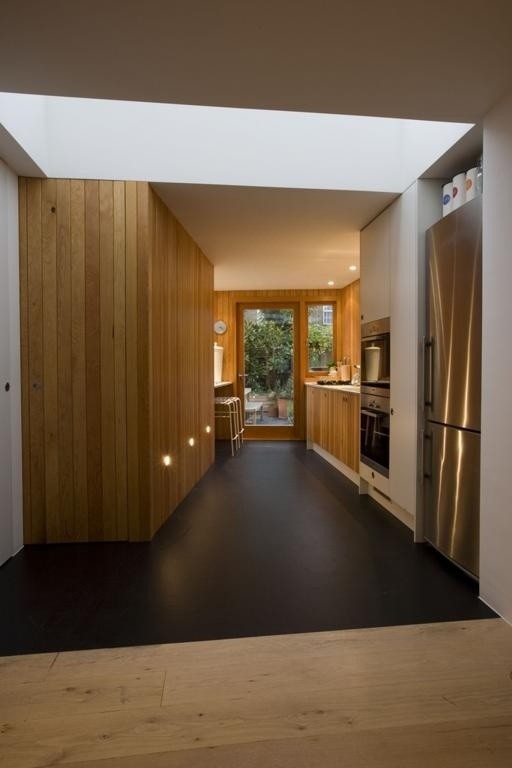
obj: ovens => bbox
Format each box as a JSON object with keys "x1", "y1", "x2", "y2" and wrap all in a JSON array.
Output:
[{"x1": 360, "y1": 393, "x2": 389, "y2": 480}]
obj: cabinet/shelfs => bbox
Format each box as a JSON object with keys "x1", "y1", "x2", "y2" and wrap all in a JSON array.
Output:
[
  {"x1": 307, "y1": 386, "x2": 360, "y2": 477},
  {"x1": 17, "y1": 178, "x2": 215, "y2": 542},
  {"x1": 360, "y1": 206, "x2": 389, "y2": 324},
  {"x1": 389, "y1": 177, "x2": 449, "y2": 543}
]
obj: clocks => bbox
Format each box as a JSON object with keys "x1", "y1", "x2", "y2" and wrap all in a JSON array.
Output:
[{"x1": 214, "y1": 319, "x2": 227, "y2": 335}]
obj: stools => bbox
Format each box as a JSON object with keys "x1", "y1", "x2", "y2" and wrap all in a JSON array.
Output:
[{"x1": 215, "y1": 396, "x2": 245, "y2": 456}]
